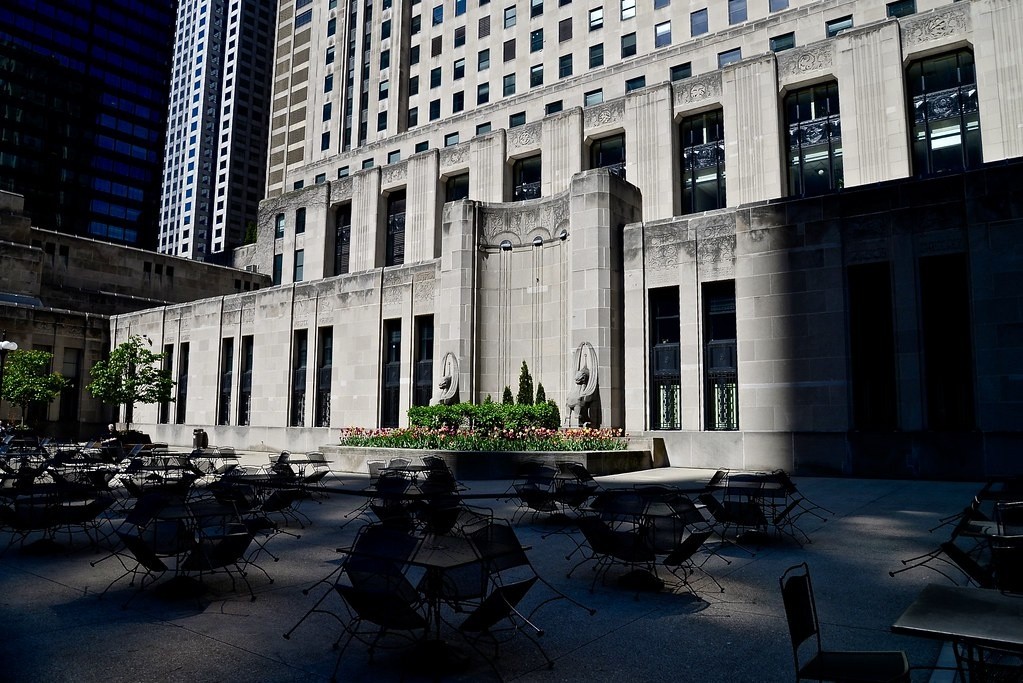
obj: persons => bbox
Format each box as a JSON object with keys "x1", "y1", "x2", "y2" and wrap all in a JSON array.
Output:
[{"x1": 100, "y1": 424, "x2": 120, "y2": 462}]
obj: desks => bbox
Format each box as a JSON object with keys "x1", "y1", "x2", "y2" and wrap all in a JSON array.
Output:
[
  {"x1": 241, "y1": 473, "x2": 306, "y2": 512},
  {"x1": 518, "y1": 474, "x2": 597, "y2": 526},
  {"x1": 140, "y1": 465, "x2": 186, "y2": 487},
  {"x1": 893, "y1": 583, "x2": 1023, "y2": 683},
  {"x1": 579, "y1": 503, "x2": 708, "y2": 564},
  {"x1": 124, "y1": 499, "x2": 261, "y2": 602},
  {"x1": 704, "y1": 481, "x2": 786, "y2": 540},
  {"x1": 334, "y1": 532, "x2": 533, "y2": 677}
]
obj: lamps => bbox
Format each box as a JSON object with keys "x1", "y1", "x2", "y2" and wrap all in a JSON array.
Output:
[{"x1": 814, "y1": 169, "x2": 824, "y2": 176}]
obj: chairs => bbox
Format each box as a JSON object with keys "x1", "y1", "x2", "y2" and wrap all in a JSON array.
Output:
[{"x1": 0, "y1": 431, "x2": 1023, "y2": 683}]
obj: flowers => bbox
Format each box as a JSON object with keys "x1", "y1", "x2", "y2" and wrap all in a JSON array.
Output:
[{"x1": 340, "y1": 425, "x2": 636, "y2": 446}]
